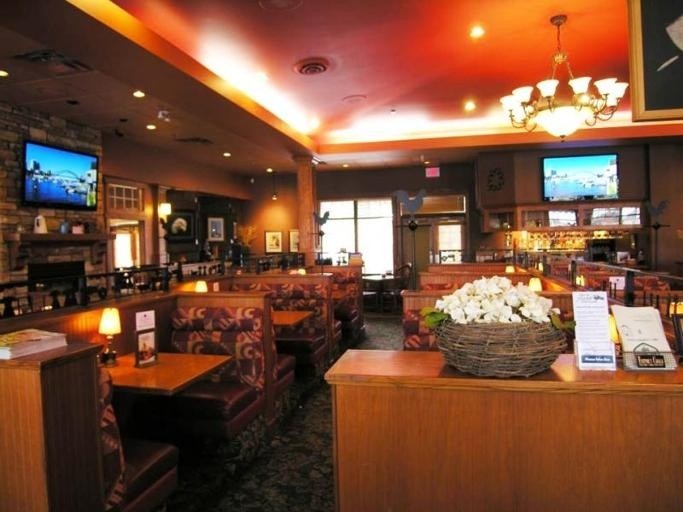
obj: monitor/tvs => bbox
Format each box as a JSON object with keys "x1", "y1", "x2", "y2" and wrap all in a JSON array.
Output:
[
  {"x1": 20, "y1": 140, "x2": 99, "y2": 212},
  {"x1": 541, "y1": 153, "x2": 621, "y2": 204}
]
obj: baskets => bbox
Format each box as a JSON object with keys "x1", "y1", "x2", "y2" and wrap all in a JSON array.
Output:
[{"x1": 435, "y1": 320, "x2": 567, "y2": 378}]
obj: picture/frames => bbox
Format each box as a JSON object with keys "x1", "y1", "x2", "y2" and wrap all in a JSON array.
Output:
[
  {"x1": 621, "y1": 0, "x2": 683, "y2": 122},
  {"x1": 265, "y1": 230, "x2": 300, "y2": 254}
]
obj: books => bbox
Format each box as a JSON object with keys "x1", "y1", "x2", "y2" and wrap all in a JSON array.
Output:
[{"x1": 0, "y1": 326, "x2": 68, "y2": 361}]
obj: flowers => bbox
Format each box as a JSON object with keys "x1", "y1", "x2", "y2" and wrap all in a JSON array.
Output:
[{"x1": 413, "y1": 274, "x2": 578, "y2": 338}]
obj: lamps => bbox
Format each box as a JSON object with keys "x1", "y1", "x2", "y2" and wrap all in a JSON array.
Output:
[{"x1": 498, "y1": 12, "x2": 631, "y2": 138}]
